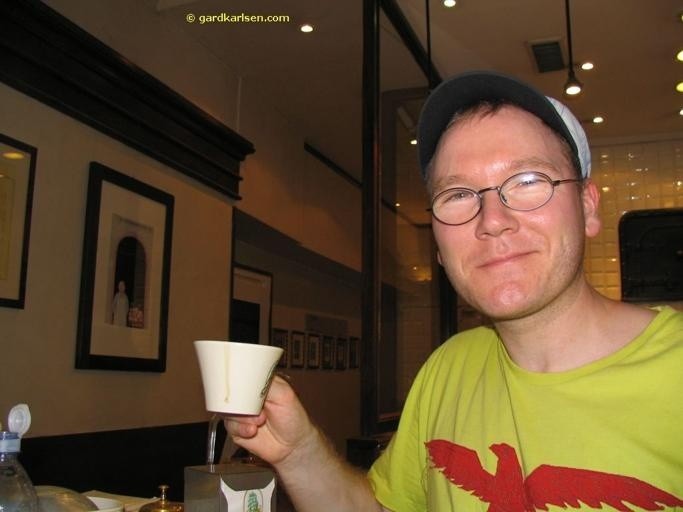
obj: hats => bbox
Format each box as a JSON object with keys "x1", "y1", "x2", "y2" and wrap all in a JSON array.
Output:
[{"x1": 416, "y1": 71, "x2": 591, "y2": 178}]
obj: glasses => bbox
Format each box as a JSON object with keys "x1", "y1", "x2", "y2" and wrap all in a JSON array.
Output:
[{"x1": 427, "y1": 171, "x2": 581, "y2": 225}]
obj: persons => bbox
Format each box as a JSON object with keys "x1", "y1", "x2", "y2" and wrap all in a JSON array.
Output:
[
  {"x1": 125, "y1": 299, "x2": 143, "y2": 329},
  {"x1": 215, "y1": 67, "x2": 683, "y2": 511},
  {"x1": 109, "y1": 277, "x2": 129, "y2": 328}
]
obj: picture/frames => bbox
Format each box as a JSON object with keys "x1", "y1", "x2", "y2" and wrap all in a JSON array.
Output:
[
  {"x1": 0, "y1": 133, "x2": 37, "y2": 310},
  {"x1": 271, "y1": 328, "x2": 288, "y2": 367},
  {"x1": 74, "y1": 161, "x2": 176, "y2": 373},
  {"x1": 336, "y1": 336, "x2": 348, "y2": 370},
  {"x1": 291, "y1": 331, "x2": 305, "y2": 367},
  {"x1": 232, "y1": 260, "x2": 271, "y2": 344},
  {"x1": 321, "y1": 334, "x2": 335, "y2": 370},
  {"x1": 350, "y1": 336, "x2": 360, "y2": 370},
  {"x1": 306, "y1": 332, "x2": 321, "y2": 368}
]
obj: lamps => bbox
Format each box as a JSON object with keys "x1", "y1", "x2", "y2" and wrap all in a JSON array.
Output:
[{"x1": 563, "y1": 0, "x2": 594, "y2": 97}]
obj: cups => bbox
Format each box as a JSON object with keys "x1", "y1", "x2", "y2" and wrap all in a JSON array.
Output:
[
  {"x1": 184, "y1": 461, "x2": 277, "y2": 512},
  {"x1": 192, "y1": 340, "x2": 285, "y2": 416}
]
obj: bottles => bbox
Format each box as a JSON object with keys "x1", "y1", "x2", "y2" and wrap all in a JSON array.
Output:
[{"x1": 0, "y1": 402, "x2": 40, "y2": 512}]
